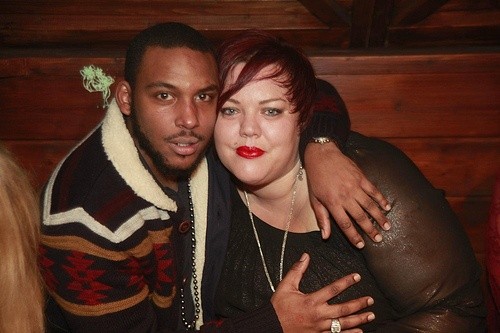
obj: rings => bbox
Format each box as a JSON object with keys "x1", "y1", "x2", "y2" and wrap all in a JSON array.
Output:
[{"x1": 331, "y1": 318, "x2": 341, "y2": 333}]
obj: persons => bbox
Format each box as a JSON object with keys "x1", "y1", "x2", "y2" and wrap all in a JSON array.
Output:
[
  {"x1": 209, "y1": 30, "x2": 487, "y2": 333},
  {"x1": 38, "y1": 23, "x2": 392, "y2": 333}
]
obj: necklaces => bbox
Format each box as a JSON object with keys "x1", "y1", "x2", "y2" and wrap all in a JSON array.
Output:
[
  {"x1": 242, "y1": 161, "x2": 303, "y2": 293},
  {"x1": 180, "y1": 174, "x2": 200, "y2": 328}
]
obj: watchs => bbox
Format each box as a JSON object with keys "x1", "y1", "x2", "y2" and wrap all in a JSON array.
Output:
[{"x1": 311, "y1": 137, "x2": 333, "y2": 144}]
